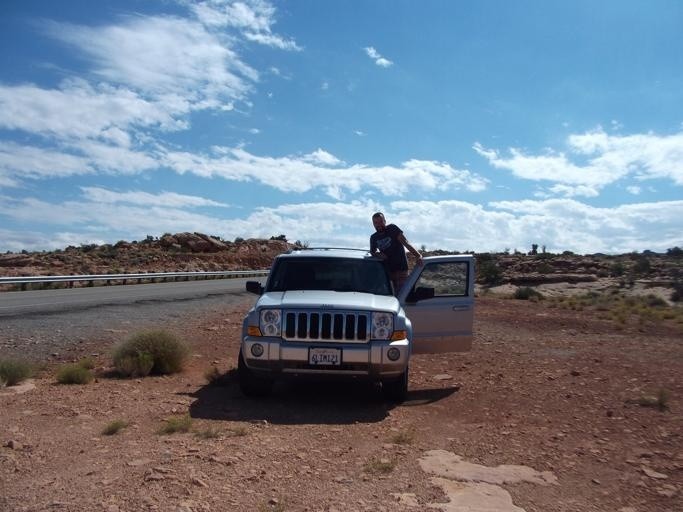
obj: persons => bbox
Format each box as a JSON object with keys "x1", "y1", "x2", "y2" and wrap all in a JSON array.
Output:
[{"x1": 369, "y1": 212, "x2": 423, "y2": 296}]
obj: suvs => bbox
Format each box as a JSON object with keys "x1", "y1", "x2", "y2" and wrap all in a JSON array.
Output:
[{"x1": 234, "y1": 242, "x2": 478, "y2": 396}]
